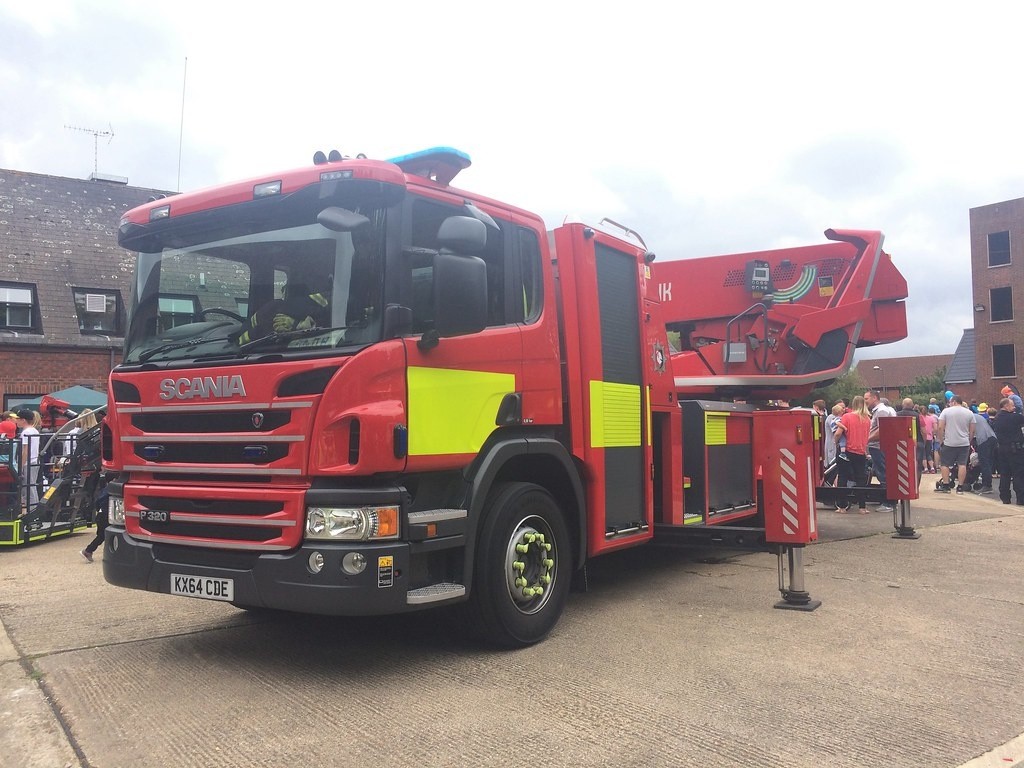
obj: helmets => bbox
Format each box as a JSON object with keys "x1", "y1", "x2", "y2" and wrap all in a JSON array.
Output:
[{"x1": 977, "y1": 403, "x2": 989, "y2": 412}]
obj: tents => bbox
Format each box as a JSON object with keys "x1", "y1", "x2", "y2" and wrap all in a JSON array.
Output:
[{"x1": 9, "y1": 382, "x2": 107, "y2": 413}]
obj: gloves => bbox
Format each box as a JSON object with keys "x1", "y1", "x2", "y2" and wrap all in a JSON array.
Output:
[{"x1": 273, "y1": 313, "x2": 316, "y2": 332}]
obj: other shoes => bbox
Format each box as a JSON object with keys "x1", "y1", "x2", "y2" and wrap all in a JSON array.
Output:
[
  {"x1": 929, "y1": 469, "x2": 935, "y2": 474},
  {"x1": 836, "y1": 508, "x2": 847, "y2": 514},
  {"x1": 978, "y1": 486, "x2": 994, "y2": 494},
  {"x1": 942, "y1": 486, "x2": 951, "y2": 493},
  {"x1": 922, "y1": 469, "x2": 929, "y2": 474},
  {"x1": 857, "y1": 509, "x2": 870, "y2": 514},
  {"x1": 839, "y1": 453, "x2": 851, "y2": 461},
  {"x1": 956, "y1": 487, "x2": 963, "y2": 494},
  {"x1": 79, "y1": 551, "x2": 95, "y2": 563},
  {"x1": 875, "y1": 506, "x2": 895, "y2": 513}
]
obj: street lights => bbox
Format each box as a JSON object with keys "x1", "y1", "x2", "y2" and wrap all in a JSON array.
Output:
[{"x1": 873, "y1": 366, "x2": 885, "y2": 399}]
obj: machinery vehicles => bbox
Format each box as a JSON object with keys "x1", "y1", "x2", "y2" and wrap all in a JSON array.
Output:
[{"x1": 99, "y1": 146, "x2": 921, "y2": 651}]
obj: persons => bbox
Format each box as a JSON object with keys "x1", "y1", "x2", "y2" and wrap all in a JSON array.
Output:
[
  {"x1": 768, "y1": 398, "x2": 792, "y2": 408},
  {"x1": 813, "y1": 392, "x2": 899, "y2": 515},
  {"x1": 236, "y1": 260, "x2": 374, "y2": 344},
  {"x1": 0, "y1": 408, "x2": 101, "y2": 532},
  {"x1": 78, "y1": 471, "x2": 122, "y2": 563},
  {"x1": 733, "y1": 397, "x2": 747, "y2": 405},
  {"x1": 892, "y1": 386, "x2": 1024, "y2": 505}
]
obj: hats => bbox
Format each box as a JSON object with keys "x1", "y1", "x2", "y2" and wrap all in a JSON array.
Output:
[
  {"x1": 1002, "y1": 387, "x2": 1015, "y2": 396},
  {"x1": 812, "y1": 399, "x2": 826, "y2": 409},
  {"x1": 8, "y1": 409, "x2": 35, "y2": 421},
  {"x1": 0, "y1": 421, "x2": 16, "y2": 439}
]
